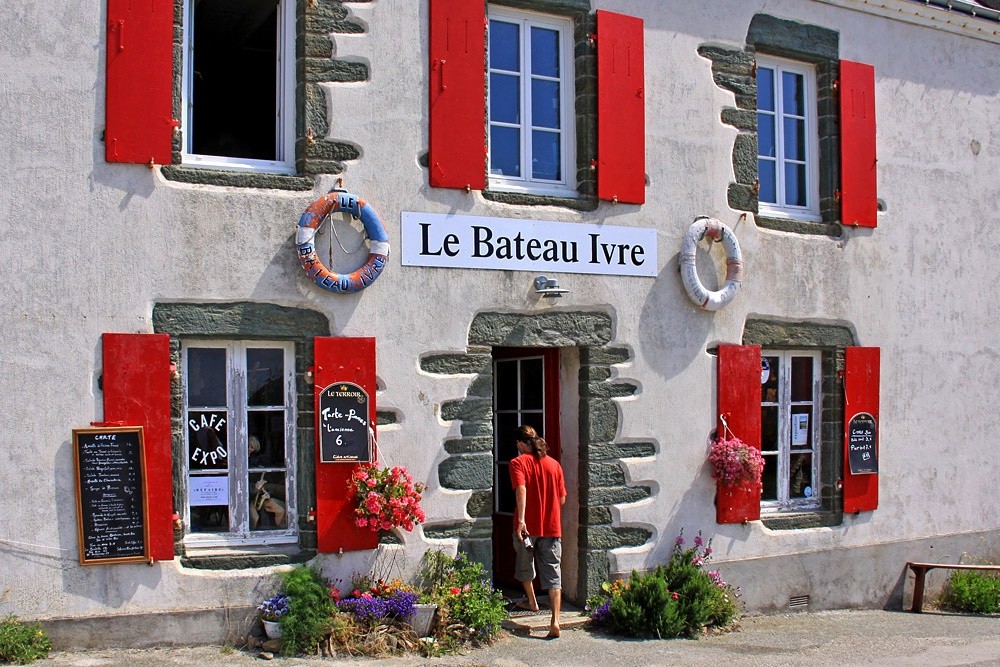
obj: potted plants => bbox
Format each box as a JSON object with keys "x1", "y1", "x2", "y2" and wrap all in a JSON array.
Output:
[{"x1": 400, "y1": 593, "x2": 437, "y2": 638}]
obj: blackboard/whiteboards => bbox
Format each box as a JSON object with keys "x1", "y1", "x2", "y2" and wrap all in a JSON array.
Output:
[
  {"x1": 848, "y1": 412, "x2": 879, "y2": 477},
  {"x1": 319, "y1": 382, "x2": 372, "y2": 465},
  {"x1": 71, "y1": 425, "x2": 153, "y2": 564}
]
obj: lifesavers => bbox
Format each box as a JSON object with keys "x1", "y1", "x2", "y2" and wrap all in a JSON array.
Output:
[
  {"x1": 681, "y1": 217, "x2": 743, "y2": 312},
  {"x1": 295, "y1": 194, "x2": 389, "y2": 293}
]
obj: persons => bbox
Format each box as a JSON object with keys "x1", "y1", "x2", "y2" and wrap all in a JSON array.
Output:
[
  {"x1": 509, "y1": 425, "x2": 567, "y2": 638},
  {"x1": 248, "y1": 434, "x2": 277, "y2": 482}
]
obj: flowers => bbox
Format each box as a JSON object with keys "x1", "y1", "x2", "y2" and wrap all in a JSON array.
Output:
[{"x1": 256, "y1": 592, "x2": 293, "y2": 618}]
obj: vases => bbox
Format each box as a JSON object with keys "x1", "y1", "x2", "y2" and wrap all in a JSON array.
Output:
[{"x1": 261, "y1": 618, "x2": 284, "y2": 639}]
